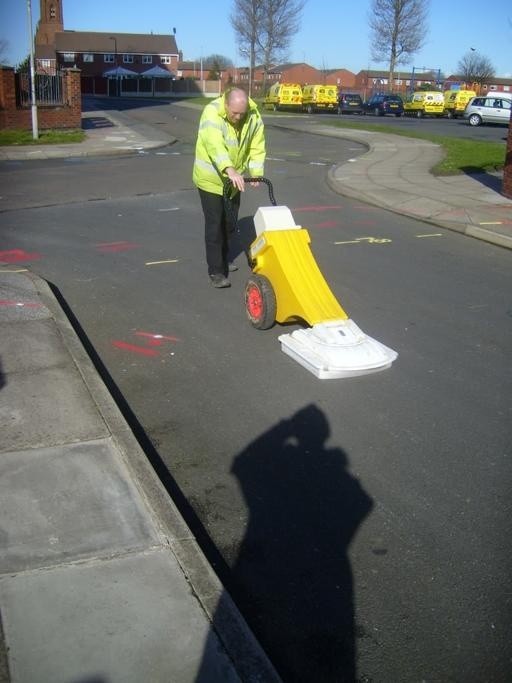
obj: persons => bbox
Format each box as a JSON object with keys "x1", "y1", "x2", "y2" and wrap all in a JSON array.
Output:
[{"x1": 191, "y1": 86, "x2": 267, "y2": 289}]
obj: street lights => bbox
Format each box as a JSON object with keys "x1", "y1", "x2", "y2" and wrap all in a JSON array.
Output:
[
  {"x1": 108, "y1": 35, "x2": 120, "y2": 96},
  {"x1": 465, "y1": 45, "x2": 477, "y2": 82}
]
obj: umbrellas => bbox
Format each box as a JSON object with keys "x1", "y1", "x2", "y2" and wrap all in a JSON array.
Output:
[
  {"x1": 102, "y1": 66, "x2": 139, "y2": 97},
  {"x1": 140, "y1": 65, "x2": 176, "y2": 96}
]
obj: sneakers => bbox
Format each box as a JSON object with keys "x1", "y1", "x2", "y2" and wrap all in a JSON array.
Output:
[
  {"x1": 229, "y1": 263, "x2": 238, "y2": 272},
  {"x1": 209, "y1": 274, "x2": 231, "y2": 288}
]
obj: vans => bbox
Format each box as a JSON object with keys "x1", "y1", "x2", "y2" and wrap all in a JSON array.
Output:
[{"x1": 260, "y1": 80, "x2": 512, "y2": 127}]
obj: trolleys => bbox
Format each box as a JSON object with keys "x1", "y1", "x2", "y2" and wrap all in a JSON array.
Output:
[{"x1": 224, "y1": 175, "x2": 403, "y2": 378}]
